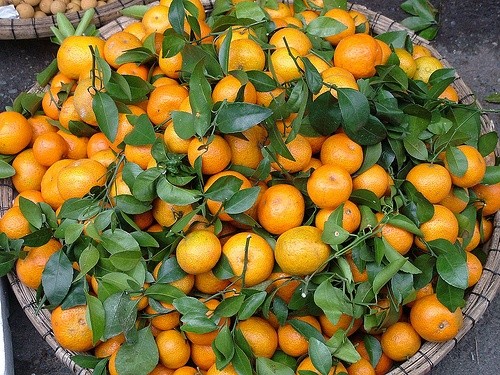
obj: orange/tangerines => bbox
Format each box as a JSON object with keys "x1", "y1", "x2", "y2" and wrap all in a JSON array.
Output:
[
  {"x1": 142, "y1": 0, "x2": 324, "y2": 34},
  {"x1": 0, "y1": 7, "x2": 500, "y2": 375}
]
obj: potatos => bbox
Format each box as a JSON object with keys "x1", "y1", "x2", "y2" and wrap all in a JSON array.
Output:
[{"x1": 0, "y1": 0, "x2": 118, "y2": 19}]
